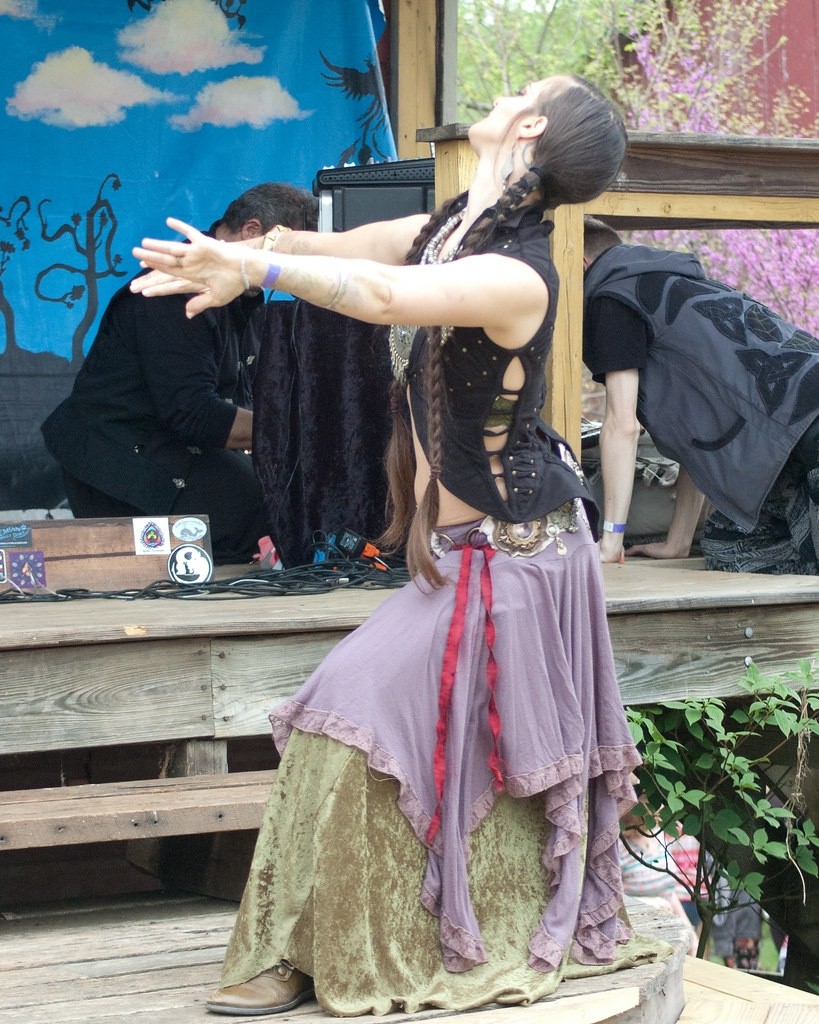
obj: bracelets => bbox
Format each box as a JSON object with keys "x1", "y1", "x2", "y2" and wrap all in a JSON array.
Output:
[
  {"x1": 263, "y1": 224, "x2": 292, "y2": 253},
  {"x1": 242, "y1": 243, "x2": 250, "y2": 291},
  {"x1": 324, "y1": 261, "x2": 347, "y2": 309},
  {"x1": 603, "y1": 520, "x2": 627, "y2": 533},
  {"x1": 262, "y1": 262, "x2": 280, "y2": 289}
]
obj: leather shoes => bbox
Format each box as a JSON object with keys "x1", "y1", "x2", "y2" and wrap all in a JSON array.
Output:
[{"x1": 207, "y1": 960, "x2": 312, "y2": 1016}]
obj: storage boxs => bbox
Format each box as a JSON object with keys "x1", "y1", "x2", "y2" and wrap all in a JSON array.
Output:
[{"x1": 0, "y1": 514, "x2": 215, "y2": 594}]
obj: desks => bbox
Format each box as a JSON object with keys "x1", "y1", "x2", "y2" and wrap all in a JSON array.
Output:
[{"x1": 414, "y1": 121, "x2": 819, "y2": 470}]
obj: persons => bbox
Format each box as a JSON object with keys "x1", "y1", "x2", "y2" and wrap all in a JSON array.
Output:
[
  {"x1": 131, "y1": 74, "x2": 672, "y2": 1016},
  {"x1": 40, "y1": 185, "x2": 320, "y2": 565},
  {"x1": 584, "y1": 216, "x2": 819, "y2": 577}
]
